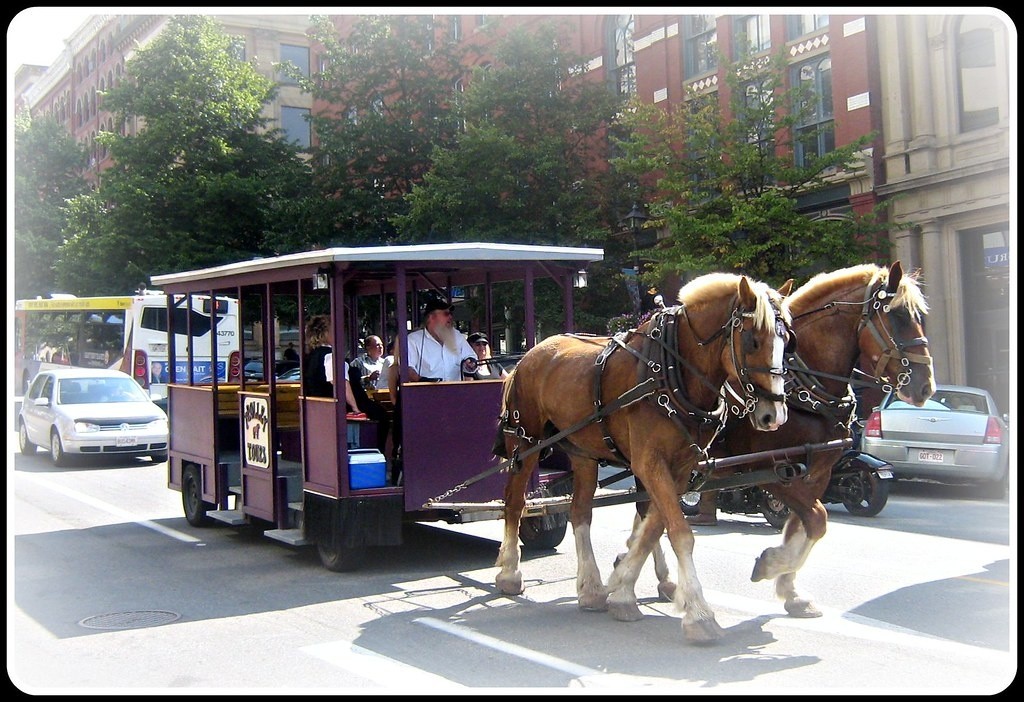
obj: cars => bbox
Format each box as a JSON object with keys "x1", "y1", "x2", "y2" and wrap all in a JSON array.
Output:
[
  {"x1": 243, "y1": 360, "x2": 302, "y2": 385},
  {"x1": 863, "y1": 383, "x2": 1009, "y2": 497},
  {"x1": 21, "y1": 368, "x2": 170, "y2": 465}
]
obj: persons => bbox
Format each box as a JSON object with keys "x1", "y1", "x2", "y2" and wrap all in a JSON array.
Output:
[
  {"x1": 350, "y1": 335, "x2": 395, "y2": 388},
  {"x1": 33, "y1": 337, "x2": 79, "y2": 366},
  {"x1": 283, "y1": 341, "x2": 300, "y2": 361},
  {"x1": 464, "y1": 332, "x2": 508, "y2": 380},
  {"x1": 303, "y1": 315, "x2": 360, "y2": 414},
  {"x1": 407, "y1": 299, "x2": 478, "y2": 382},
  {"x1": 385, "y1": 335, "x2": 400, "y2": 486}
]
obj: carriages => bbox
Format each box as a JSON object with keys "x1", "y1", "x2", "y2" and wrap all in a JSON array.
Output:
[{"x1": 152, "y1": 238, "x2": 939, "y2": 652}]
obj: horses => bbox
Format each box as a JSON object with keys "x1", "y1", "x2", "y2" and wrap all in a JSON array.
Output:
[{"x1": 488, "y1": 257, "x2": 938, "y2": 647}]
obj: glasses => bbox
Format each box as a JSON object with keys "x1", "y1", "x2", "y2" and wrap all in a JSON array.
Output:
[{"x1": 473, "y1": 343, "x2": 487, "y2": 346}]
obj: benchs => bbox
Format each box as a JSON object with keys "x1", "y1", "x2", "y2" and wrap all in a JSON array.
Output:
[{"x1": 199, "y1": 384, "x2": 393, "y2": 502}]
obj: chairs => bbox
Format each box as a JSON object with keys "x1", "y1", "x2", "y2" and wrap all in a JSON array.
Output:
[{"x1": 63, "y1": 382, "x2": 85, "y2": 403}]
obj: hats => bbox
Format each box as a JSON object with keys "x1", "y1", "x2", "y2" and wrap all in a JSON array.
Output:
[
  {"x1": 467, "y1": 332, "x2": 488, "y2": 343},
  {"x1": 425, "y1": 299, "x2": 457, "y2": 317}
]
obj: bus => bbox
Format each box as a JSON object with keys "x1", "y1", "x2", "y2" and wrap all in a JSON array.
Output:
[{"x1": 14, "y1": 293, "x2": 243, "y2": 414}]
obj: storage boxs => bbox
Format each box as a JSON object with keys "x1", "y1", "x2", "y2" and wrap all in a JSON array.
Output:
[{"x1": 347, "y1": 448, "x2": 387, "y2": 491}]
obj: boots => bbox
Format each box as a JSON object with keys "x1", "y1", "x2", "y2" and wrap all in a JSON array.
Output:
[{"x1": 686, "y1": 491, "x2": 718, "y2": 526}]
